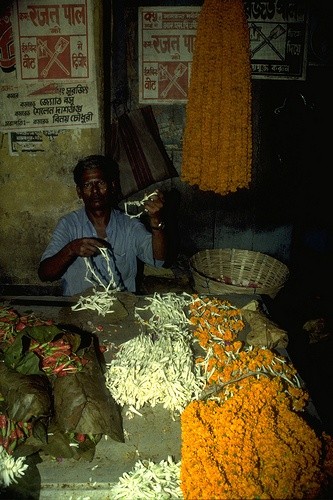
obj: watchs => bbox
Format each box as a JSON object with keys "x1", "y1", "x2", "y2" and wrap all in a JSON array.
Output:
[{"x1": 151, "y1": 219, "x2": 165, "y2": 231}]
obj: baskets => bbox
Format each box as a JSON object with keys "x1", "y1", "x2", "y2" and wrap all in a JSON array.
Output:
[{"x1": 189, "y1": 248, "x2": 289, "y2": 301}]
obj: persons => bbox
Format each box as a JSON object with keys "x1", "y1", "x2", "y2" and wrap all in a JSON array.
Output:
[{"x1": 37, "y1": 154, "x2": 167, "y2": 296}]
